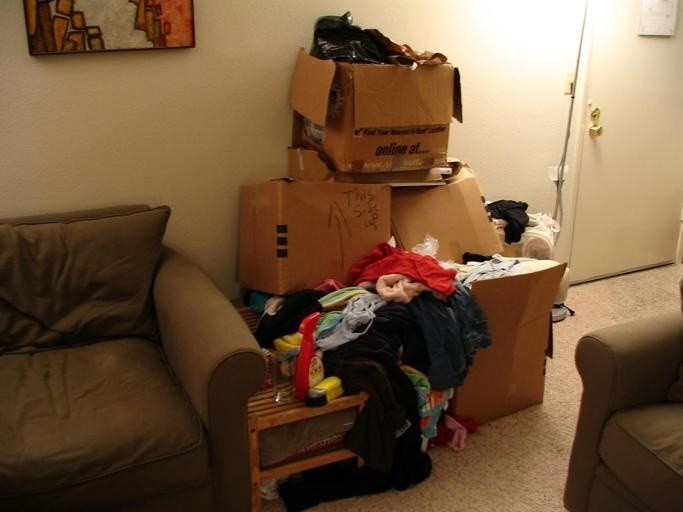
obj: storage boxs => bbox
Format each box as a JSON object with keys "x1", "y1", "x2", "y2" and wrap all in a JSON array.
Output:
[
  {"x1": 447, "y1": 260, "x2": 567, "y2": 432},
  {"x1": 285, "y1": 46, "x2": 465, "y2": 174},
  {"x1": 237, "y1": 179, "x2": 393, "y2": 294},
  {"x1": 390, "y1": 156, "x2": 505, "y2": 263}
]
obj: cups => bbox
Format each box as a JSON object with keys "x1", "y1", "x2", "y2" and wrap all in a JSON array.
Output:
[{"x1": 268, "y1": 352, "x2": 294, "y2": 403}]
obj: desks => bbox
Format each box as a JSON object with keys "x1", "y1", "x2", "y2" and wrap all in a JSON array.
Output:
[{"x1": 236, "y1": 306, "x2": 371, "y2": 511}]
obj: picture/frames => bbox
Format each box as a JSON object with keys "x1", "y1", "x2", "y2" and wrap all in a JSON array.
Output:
[{"x1": 22, "y1": 0, "x2": 196, "y2": 57}]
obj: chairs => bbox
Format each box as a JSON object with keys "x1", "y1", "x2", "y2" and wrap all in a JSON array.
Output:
[
  {"x1": 0, "y1": 204, "x2": 270, "y2": 512},
  {"x1": 563, "y1": 279, "x2": 682, "y2": 511}
]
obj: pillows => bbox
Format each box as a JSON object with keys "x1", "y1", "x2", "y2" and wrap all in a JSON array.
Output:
[{"x1": 0, "y1": 204, "x2": 173, "y2": 356}]
